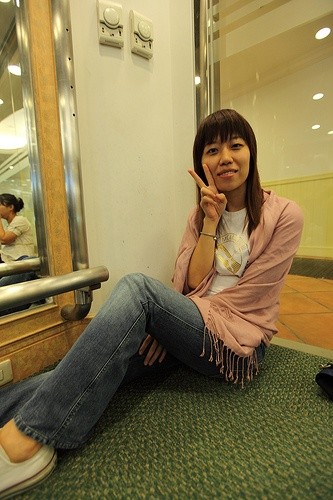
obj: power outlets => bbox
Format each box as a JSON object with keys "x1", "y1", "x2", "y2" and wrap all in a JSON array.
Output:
[
  {"x1": 129, "y1": 10, "x2": 154, "y2": 59},
  {"x1": 0, "y1": 359, "x2": 15, "y2": 388},
  {"x1": 96, "y1": 0, "x2": 125, "y2": 48}
]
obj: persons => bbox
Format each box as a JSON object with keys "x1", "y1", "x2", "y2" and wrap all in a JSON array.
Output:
[
  {"x1": 0, "y1": 193, "x2": 46, "y2": 319},
  {"x1": 0, "y1": 109, "x2": 304, "y2": 500}
]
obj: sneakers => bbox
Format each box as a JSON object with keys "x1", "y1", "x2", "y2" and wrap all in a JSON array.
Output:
[{"x1": 0, "y1": 446, "x2": 58, "y2": 500}]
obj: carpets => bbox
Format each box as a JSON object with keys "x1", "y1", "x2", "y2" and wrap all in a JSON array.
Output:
[{"x1": 0, "y1": 333, "x2": 332, "y2": 500}]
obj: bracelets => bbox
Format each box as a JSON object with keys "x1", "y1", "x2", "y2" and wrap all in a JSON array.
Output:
[{"x1": 200, "y1": 232, "x2": 219, "y2": 240}]
